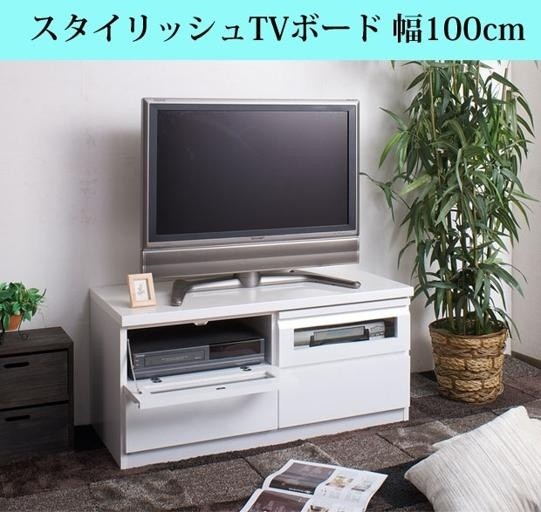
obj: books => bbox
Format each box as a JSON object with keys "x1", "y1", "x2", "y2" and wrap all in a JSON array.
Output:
[{"x1": 239, "y1": 458, "x2": 387, "y2": 512}]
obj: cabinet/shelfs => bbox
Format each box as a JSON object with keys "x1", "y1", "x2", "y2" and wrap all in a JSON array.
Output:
[
  {"x1": 0, "y1": 326, "x2": 74, "y2": 467},
  {"x1": 90, "y1": 268, "x2": 415, "y2": 471}
]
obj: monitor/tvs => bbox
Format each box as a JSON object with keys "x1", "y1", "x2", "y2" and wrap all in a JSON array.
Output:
[{"x1": 140, "y1": 97, "x2": 361, "y2": 307}]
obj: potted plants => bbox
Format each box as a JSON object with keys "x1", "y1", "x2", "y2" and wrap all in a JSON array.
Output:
[
  {"x1": 360, "y1": 60, "x2": 539, "y2": 405},
  {"x1": 0, "y1": 281, "x2": 46, "y2": 340}
]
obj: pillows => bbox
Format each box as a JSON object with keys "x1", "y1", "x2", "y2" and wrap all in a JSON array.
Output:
[
  {"x1": 432, "y1": 417, "x2": 541, "y2": 453},
  {"x1": 403, "y1": 407, "x2": 541, "y2": 511}
]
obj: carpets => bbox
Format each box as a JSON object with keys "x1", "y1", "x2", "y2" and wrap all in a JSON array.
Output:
[{"x1": 168, "y1": 453, "x2": 433, "y2": 512}]
